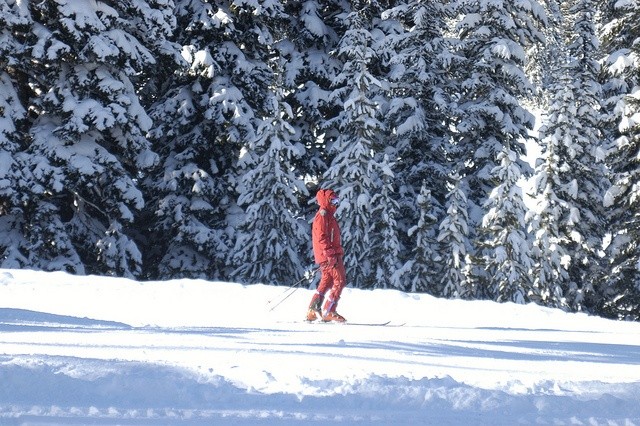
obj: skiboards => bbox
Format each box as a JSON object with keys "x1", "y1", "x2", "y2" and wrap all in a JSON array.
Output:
[{"x1": 293, "y1": 321, "x2": 404, "y2": 326}]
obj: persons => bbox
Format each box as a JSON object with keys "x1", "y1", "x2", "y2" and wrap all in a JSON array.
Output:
[{"x1": 306, "y1": 189, "x2": 346, "y2": 323}]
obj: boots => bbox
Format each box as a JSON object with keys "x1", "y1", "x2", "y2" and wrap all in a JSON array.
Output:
[
  {"x1": 322, "y1": 300, "x2": 345, "y2": 321},
  {"x1": 307, "y1": 293, "x2": 325, "y2": 320}
]
